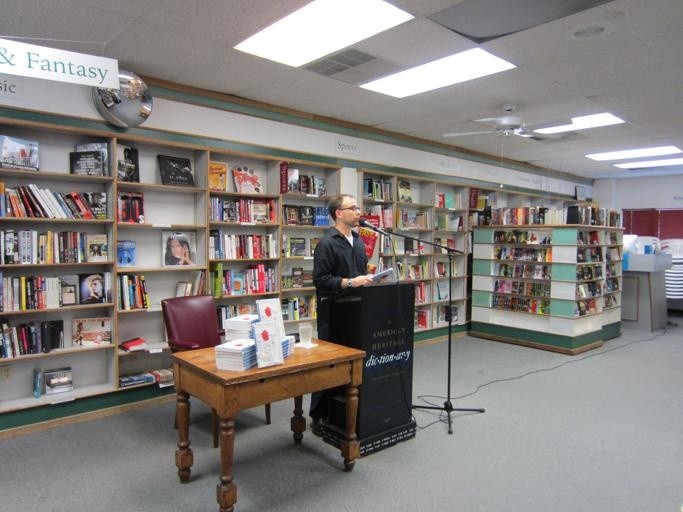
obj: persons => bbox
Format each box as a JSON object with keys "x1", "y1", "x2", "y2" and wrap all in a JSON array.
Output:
[{"x1": 308, "y1": 194, "x2": 388, "y2": 438}]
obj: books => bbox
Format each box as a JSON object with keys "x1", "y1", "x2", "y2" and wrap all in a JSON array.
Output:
[
  {"x1": 281, "y1": 294, "x2": 318, "y2": 321},
  {"x1": 437, "y1": 281, "x2": 450, "y2": 300},
  {"x1": 414, "y1": 308, "x2": 430, "y2": 330},
  {"x1": 577, "y1": 229, "x2": 600, "y2": 244},
  {"x1": 576, "y1": 263, "x2": 602, "y2": 280},
  {"x1": 493, "y1": 228, "x2": 553, "y2": 244},
  {"x1": 282, "y1": 233, "x2": 322, "y2": 257},
  {"x1": 7, "y1": 183, "x2": 108, "y2": 219},
  {"x1": 605, "y1": 263, "x2": 617, "y2": 276},
  {"x1": 0, "y1": 134, "x2": 40, "y2": 172},
  {"x1": 214, "y1": 312, "x2": 258, "y2": 372},
  {"x1": 403, "y1": 237, "x2": 426, "y2": 255},
  {"x1": 117, "y1": 191, "x2": 145, "y2": 224},
  {"x1": 575, "y1": 280, "x2": 602, "y2": 298},
  {"x1": 192, "y1": 269, "x2": 206, "y2": 296},
  {"x1": 369, "y1": 267, "x2": 398, "y2": 285},
  {"x1": 147, "y1": 368, "x2": 174, "y2": 383},
  {"x1": 605, "y1": 247, "x2": 620, "y2": 262},
  {"x1": 397, "y1": 179, "x2": 413, "y2": 204},
  {"x1": 163, "y1": 321, "x2": 171, "y2": 343},
  {"x1": 467, "y1": 231, "x2": 473, "y2": 255},
  {"x1": 117, "y1": 143, "x2": 139, "y2": 183},
  {"x1": 468, "y1": 187, "x2": 494, "y2": 210},
  {"x1": 575, "y1": 298, "x2": 597, "y2": 317},
  {"x1": 32, "y1": 368, "x2": 42, "y2": 399},
  {"x1": 46, "y1": 319, "x2": 66, "y2": 349},
  {"x1": 217, "y1": 303, "x2": 256, "y2": 329},
  {"x1": 605, "y1": 278, "x2": 619, "y2": 292},
  {"x1": 280, "y1": 163, "x2": 327, "y2": 196},
  {"x1": 209, "y1": 262, "x2": 277, "y2": 297},
  {"x1": 86, "y1": 234, "x2": 108, "y2": 263},
  {"x1": 105, "y1": 271, "x2": 114, "y2": 304},
  {"x1": 71, "y1": 316, "x2": 112, "y2": 348},
  {"x1": 157, "y1": 155, "x2": 196, "y2": 187},
  {"x1": 604, "y1": 229, "x2": 618, "y2": 244},
  {"x1": 363, "y1": 175, "x2": 393, "y2": 201},
  {"x1": 414, "y1": 282, "x2": 427, "y2": 305},
  {"x1": 494, "y1": 277, "x2": 551, "y2": 297},
  {"x1": 433, "y1": 237, "x2": 457, "y2": 254},
  {"x1": 493, "y1": 260, "x2": 552, "y2": 279},
  {"x1": 76, "y1": 142, "x2": 109, "y2": 177},
  {"x1": 469, "y1": 200, "x2": 624, "y2": 228},
  {"x1": 492, "y1": 293, "x2": 550, "y2": 314},
  {"x1": 208, "y1": 160, "x2": 228, "y2": 192},
  {"x1": 116, "y1": 274, "x2": 151, "y2": 310},
  {"x1": 252, "y1": 319, "x2": 284, "y2": 369},
  {"x1": 0, "y1": 316, "x2": 49, "y2": 359},
  {"x1": 395, "y1": 206, "x2": 434, "y2": 230},
  {"x1": 209, "y1": 229, "x2": 277, "y2": 259},
  {"x1": 176, "y1": 281, "x2": 193, "y2": 298},
  {"x1": 602, "y1": 293, "x2": 617, "y2": 309},
  {"x1": 0, "y1": 270, "x2": 63, "y2": 313},
  {"x1": 121, "y1": 336, "x2": 148, "y2": 352},
  {"x1": 577, "y1": 247, "x2": 602, "y2": 262},
  {"x1": 232, "y1": 166, "x2": 265, "y2": 193},
  {"x1": 280, "y1": 335, "x2": 296, "y2": 359},
  {"x1": 434, "y1": 257, "x2": 458, "y2": 278},
  {"x1": 395, "y1": 258, "x2": 431, "y2": 279},
  {"x1": 362, "y1": 203, "x2": 392, "y2": 228},
  {"x1": 116, "y1": 240, "x2": 136, "y2": 267},
  {"x1": 434, "y1": 213, "x2": 464, "y2": 232},
  {"x1": 255, "y1": 297, "x2": 287, "y2": 339},
  {"x1": 161, "y1": 231, "x2": 198, "y2": 267},
  {"x1": 0, "y1": 182, "x2": 6, "y2": 217},
  {"x1": 79, "y1": 272, "x2": 105, "y2": 305},
  {"x1": 43, "y1": 367, "x2": 74, "y2": 395},
  {"x1": 146, "y1": 341, "x2": 170, "y2": 351},
  {"x1": 433, "y1": 303, "x2": 460, "y2": 325},
  {"x1": 436, "y1": 189, "x2": 466, "y2": 209},
  {"x1": 61, "y1": 284, "x2": 77, "y2": 306},
  {"x1": 119, "y1": 371, "x2": 155, "y2": 388},
  {"x1": 282, "y1": 204, "x2": 332, "y2": 226},
  {"x1": 210, "y1": 194, "x2": 277, "y2": 223},
  {"x1": 0, "y1": 229, "x2": 87, "y2": 265},
  {"x1": 492, "y1": 246, "x2": 552, "y2": 262},
  {"x1": 378, "y1": 257, "x2": 391, "y2": 271},
  {"x1": 380, "y1": 234, "x2": 398, "y2": 256},
  {"x1": 118, "y1": 344, "x2": 146, "y2": 353},
  {"x1": 69, "y1": 150, "x2": 103, "y2": 177},
  {"x1": 281, "y1": 264, "x2": 315, "y2": 289}
]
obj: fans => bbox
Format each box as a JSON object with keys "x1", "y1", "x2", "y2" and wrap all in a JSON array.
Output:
[{"x1": 441, "y1": 104, "x2": 572, "y2": 146}]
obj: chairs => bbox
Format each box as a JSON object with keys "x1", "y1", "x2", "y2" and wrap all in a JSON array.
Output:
[
  {"x1": 160, "y1": 294, "x2": 270, "y2": 448},
  {"x1": 281, "y1": 157, "x2": 340, "y2": 339}
]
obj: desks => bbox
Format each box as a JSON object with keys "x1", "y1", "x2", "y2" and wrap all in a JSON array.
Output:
[
  {"x1": 138, "y1": 105, "x2": 151, "y2": 120},
  {"x1": 168, "y1": 338, "x2": 366, "y2": 510}
]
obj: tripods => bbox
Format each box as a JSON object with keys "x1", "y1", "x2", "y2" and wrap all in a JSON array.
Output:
[{"x1": 412, "y1": 252, "x2": 485, "y2": 434}]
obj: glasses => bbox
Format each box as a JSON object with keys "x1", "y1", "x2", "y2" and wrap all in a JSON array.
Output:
[{"x1": 335, "y1": 205, "x2": 360, "y2": 212}]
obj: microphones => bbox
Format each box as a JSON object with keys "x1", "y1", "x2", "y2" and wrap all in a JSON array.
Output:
[{"x1": 359, "y1": 217, "x2": 388, "y2": 236}]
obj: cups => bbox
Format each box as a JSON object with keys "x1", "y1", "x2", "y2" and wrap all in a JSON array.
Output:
[{"x1": 298, "y1": 322, "x2": 312, "y2": 346}]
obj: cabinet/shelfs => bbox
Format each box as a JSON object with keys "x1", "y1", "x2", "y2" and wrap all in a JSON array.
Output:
[
  {"x1": 433, "y1": 180, "x2": 466, "y2": 329},
  {"x1": 467, "y1": 223, "x2": 624, "y2": 356},
  {"x1": 358, "y1": 167, "x2": 395, "y2": 282},
  {"x1": 112, "y1": 77, "x2": 143, "y2": 101},
  {"x1": 208, "y1": 146, "x2": 282, "y2": 334},
  {"x1": 116, "y1": 133, "x2": 208, "y2": 390},
  {"x1": 466, "y1": 184, "x2": 590, "y2": 223},
  {"x1": 396, "y1": 174, "x2": 434, "y2": 332},
  {"x1": 0, "y1": 117, "x2": 115, "y2": 414},
  {"x1": 92, "y1": 87, "x2": 128, "y2": 128}
]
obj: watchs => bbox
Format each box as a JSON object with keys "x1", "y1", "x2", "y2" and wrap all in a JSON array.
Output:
[{"x1": 347, "y1": 278, "x2": 353, "y2": 289}]
